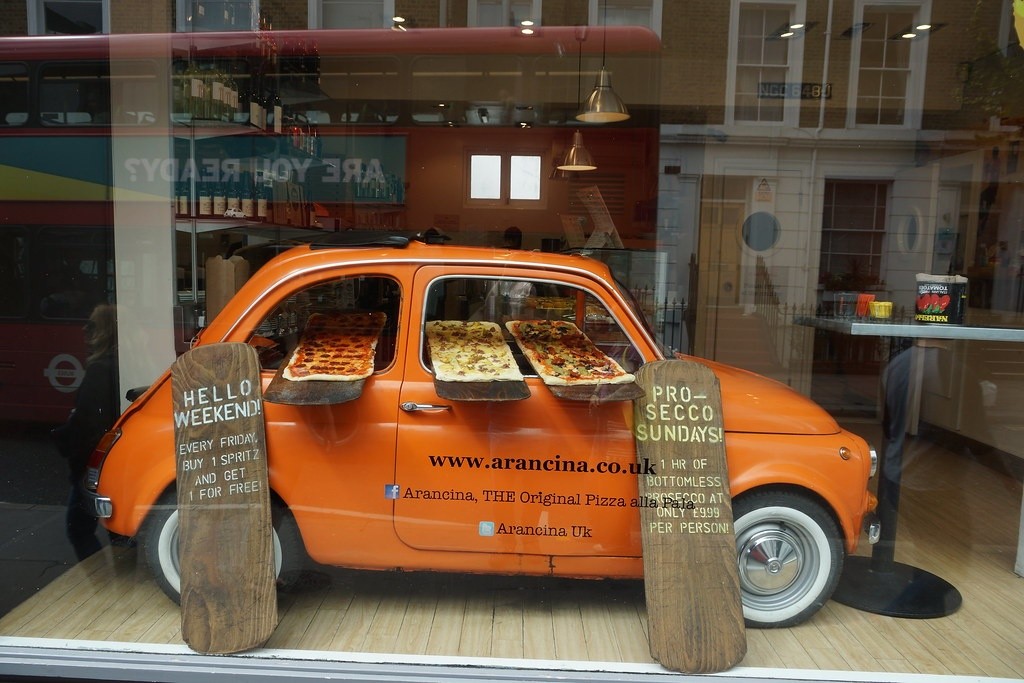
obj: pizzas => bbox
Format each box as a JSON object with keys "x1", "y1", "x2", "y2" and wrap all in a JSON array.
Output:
[
  {"x1": 283, "y1": 312, "x2": 388, "y2": 382},
  {"x1": 505, "y1": 321, "x2": 636, "y2": 386},
  {"x1": 424, "y1": 321, "x2": 523, "y2": 383}
]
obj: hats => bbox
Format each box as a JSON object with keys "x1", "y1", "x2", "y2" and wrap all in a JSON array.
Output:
[{"x1": 423, "y1": 225, "x2": 454, "y2": 242}]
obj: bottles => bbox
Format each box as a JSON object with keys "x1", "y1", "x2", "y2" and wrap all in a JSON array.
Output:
[{"x1": 166, "y1": 0, "x2": 416, "y2": 236}]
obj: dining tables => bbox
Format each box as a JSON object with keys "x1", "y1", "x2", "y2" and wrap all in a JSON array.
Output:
[{"x1": 795, "y1": 310, "x2": 1023, "y2": 620}]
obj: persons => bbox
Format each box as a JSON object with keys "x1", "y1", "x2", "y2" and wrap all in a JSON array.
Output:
[
  {"x1": 934, "y1": 213, "x2": 1024, "y2": 312},
  {"x1": 47, "y1": 305, "x2": 136, "y2": 621},
  {"x1": 876, "y1": 320, "x2": 1023, "y2": 617},
  {"x1": 422, "y1": 225, "x2": 525, "y2": 249}
]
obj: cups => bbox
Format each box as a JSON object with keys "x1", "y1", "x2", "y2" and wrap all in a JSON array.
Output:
[
  {"x1": 834, "y1": 290, "x2": 858, "y2": 317},
  {"x1": 868, "y1": 300, "x2": 895, "y2": 321}
]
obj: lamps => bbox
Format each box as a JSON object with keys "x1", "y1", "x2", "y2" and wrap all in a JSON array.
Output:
[
  {"x1": 768, "y1": 19, "x2": 946, "y2": 44},
  {"x1": 554, "y1": 24, "x2": 599, "y2": 172},
  {"x1": 387, "y1": 9, "x2": 538, "y2": 39},
  {"x1": 575, "y1": 0, "x2": 631, "y2": 124}
]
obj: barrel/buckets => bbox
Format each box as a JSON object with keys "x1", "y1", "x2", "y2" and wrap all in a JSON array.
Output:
[{"x1": 916, "y1": 273, "x2": 968, "y2": 326}]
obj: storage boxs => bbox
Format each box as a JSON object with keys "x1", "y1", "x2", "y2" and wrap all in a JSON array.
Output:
[{"x1": 914, "y1": 272, "x2": 968, "y2": 326}]
